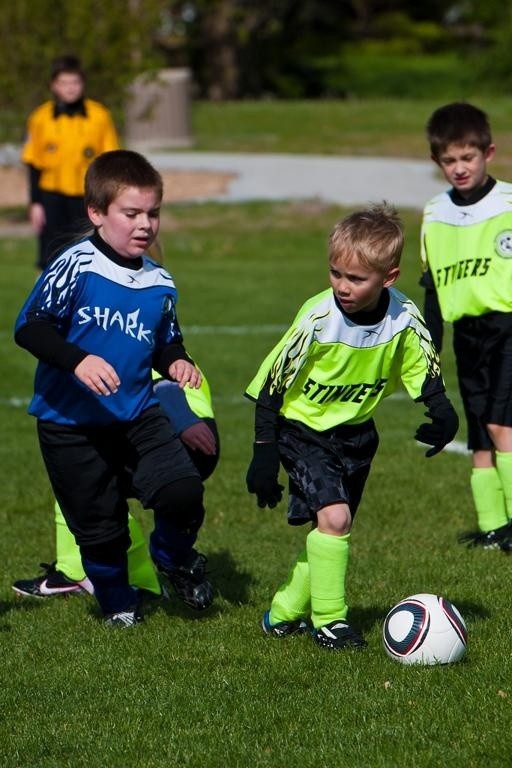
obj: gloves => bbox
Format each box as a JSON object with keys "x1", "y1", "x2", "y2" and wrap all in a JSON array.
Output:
[
  {"x1": 415, "y1": 400, "x2": 458, "y2": 456},
  {"x1": 246, "y1": 440, "x2": 285, "y2": 509}
]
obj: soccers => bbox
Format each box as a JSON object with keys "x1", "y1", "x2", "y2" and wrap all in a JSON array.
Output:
[{"x1": 383, "y1": 594, "x2": 467, "y2": 665}]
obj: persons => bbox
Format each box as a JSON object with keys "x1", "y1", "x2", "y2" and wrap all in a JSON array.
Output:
[
  {"x1": 15, "y1": 148, "x2": 214, "y2": 627},
  {"x1": 21, "y1": 54, "x2": 122, "y2": 271},
  {"x1": 417, "y1": 102, "x2": 512, "y2": 555},
  {"x1": 11, "y1": 352, "x2": 220, "y2": 614},
  {"x1": 244, "y1": 197, "x2": 459, "y2": 651}
]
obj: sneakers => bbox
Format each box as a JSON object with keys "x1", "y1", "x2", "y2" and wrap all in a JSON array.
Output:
[
  {"x1": 262, "y1": 609, "x2": 312, "y2": 638},
  {"x1": 456, "y1": 522, "x2": 511, "y2": 553},
  {"x1": 12, "y1": 561, "x2": 95, "y2": 596},
  {"x1": 313, "y1": 617, "x2": 368, "y2": 648},
  {"x1": 147, "y1": 533, "x2": 213, "y2": 610},
  {"x1": 110, "y1": 607, "x2": 144, "y2": 630}
]
